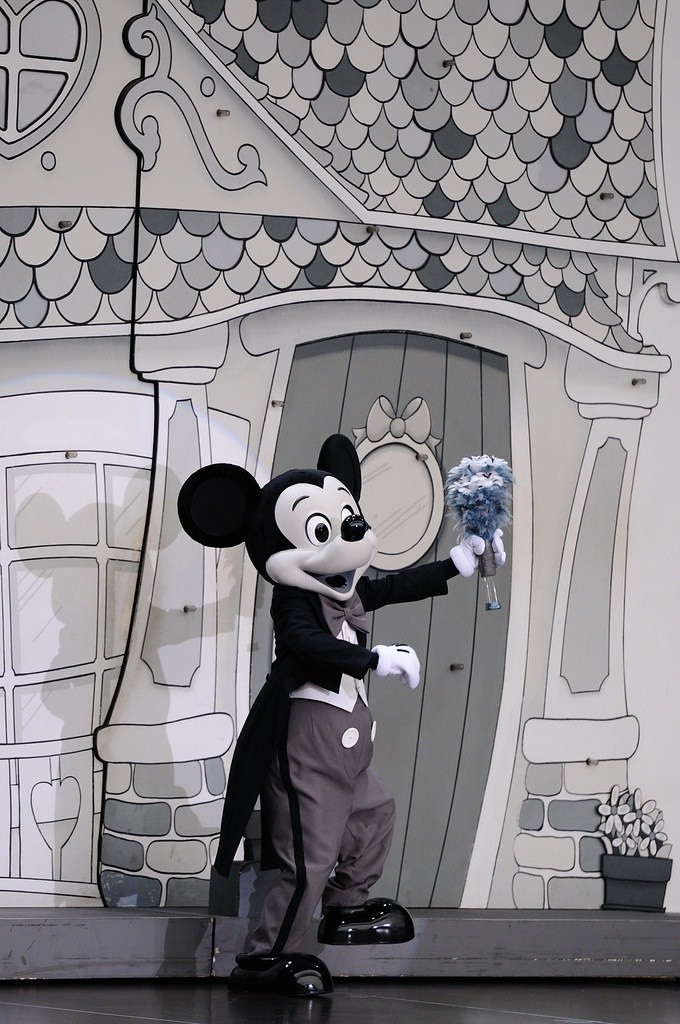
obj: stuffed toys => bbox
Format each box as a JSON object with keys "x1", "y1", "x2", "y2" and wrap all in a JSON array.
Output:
[{"x1": 177, "y1": 434, "x2": 507, "y2": 1024}]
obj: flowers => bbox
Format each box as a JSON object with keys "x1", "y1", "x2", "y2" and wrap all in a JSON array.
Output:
[{"x1": 438, "y1": 455, "x2": 514, "y2": 543}]
